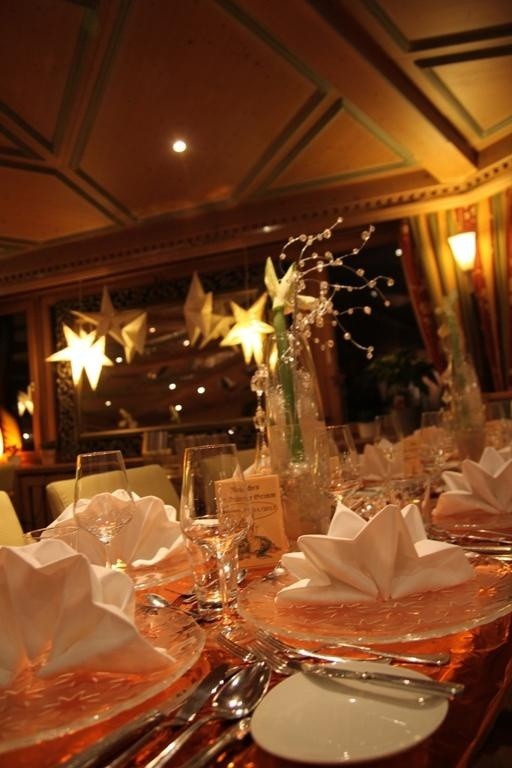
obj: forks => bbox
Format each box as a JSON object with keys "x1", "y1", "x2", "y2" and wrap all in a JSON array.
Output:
[{"x1": 214, "y1": 625, "x2": 465, "y2": 702}]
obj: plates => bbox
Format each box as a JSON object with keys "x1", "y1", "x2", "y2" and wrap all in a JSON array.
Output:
[{"x1": 250, "y1": 662, "x2": 451, "y2": 765}]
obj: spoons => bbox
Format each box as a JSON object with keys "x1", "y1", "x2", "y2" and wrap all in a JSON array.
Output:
[{"x1": 142, "y1": 663, "x2": 272, "y2": 767}]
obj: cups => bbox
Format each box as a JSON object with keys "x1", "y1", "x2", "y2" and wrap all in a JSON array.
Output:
[{"x1": 22, "y1": 526, "x2": 77, "y2": 553}]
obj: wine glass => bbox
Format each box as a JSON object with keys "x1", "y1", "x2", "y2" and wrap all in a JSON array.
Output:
[
  {"x1": 312, "y1": 413, "x2": 459, "y2": 523},
  {"x1": 73, "y1": 444, "x2": 252, "y2": 647}
]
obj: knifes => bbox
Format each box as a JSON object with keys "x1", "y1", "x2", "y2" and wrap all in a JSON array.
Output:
[{"x1": 55, "y1": 662, "x2": 227, "y2": 768}]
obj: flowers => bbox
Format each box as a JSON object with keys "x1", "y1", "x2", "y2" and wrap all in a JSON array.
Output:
[
  {"x1": 437, "y1": 294, "x2": 471, "y2": 417},
  {"x1": 264, "y1": 218, "x2": 387, "y2": 461}
]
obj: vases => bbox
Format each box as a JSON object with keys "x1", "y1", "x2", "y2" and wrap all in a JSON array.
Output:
[
  {"x1": 449, "y1": 362, "x2": 483, "y2": 462},
  {"x1": 261, "y1": 333, "x2": 340, "y2": 546}
]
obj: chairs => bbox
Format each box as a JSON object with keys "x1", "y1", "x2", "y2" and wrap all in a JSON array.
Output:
[
  {"x1": 41, "y1": 465, "x2": 183, "y2": 520},
  {"x1": 0, "y1": 491, "x2": 25, "y2": 547},
  {"x1": 203, "y1": 448, "x2": 257, "y2": 514}
]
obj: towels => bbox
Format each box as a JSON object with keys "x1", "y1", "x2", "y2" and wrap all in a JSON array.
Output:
[
  {"x1": 432, "y1": 440, "x2": 512, "y2": 518},
  {"x1": 354, "y1": 436, "x2": 403, "y2": 477},
  {"x1": 38, "y1": 486, "x2": 184, "y2": 569},
  {"x1": 0, "y1": 539, "x2": 171, "y2": 690},
  {"x1": 14, "y1": 457, "x2": 147, "y2": 543},
  {"x1": 274, "y1": 501, "x2": 474, "y2": 606}
]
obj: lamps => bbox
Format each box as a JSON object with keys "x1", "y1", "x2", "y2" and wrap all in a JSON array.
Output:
[{"x1": 448, "y1": 232, "x2": 494, "y2": 392}]
